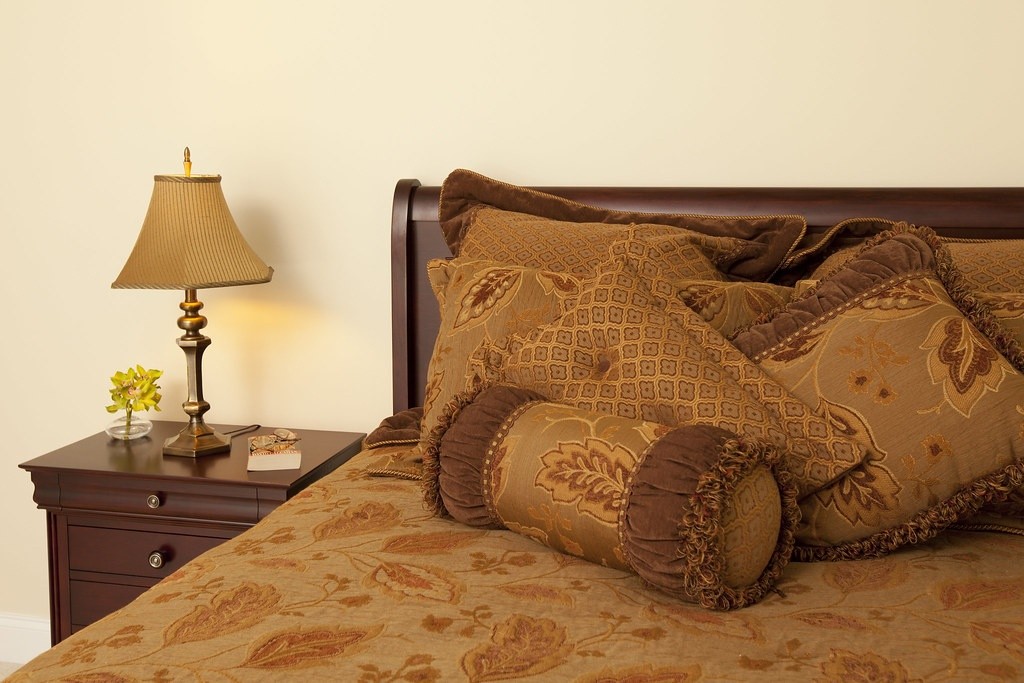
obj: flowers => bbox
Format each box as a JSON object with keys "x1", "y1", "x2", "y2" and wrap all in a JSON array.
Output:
[{"x1": 106, "y1": 369, "x2": 165, "y2": 430}]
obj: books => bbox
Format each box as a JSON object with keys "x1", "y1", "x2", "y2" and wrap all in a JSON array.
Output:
[{"x1": 246, "y1": 435, "x2": 301, "y2": 471}]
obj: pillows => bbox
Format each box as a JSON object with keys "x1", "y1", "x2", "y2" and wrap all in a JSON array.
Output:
[
  {"x1": 362, "y1": 171, "x2": 806, "y2": 453},
  {"x1": 730, "y1": 225, "x2": 1024, "y2": 560},
  {"x1": 366, "y1": 256, "x2": 820, "y2": 481},
  {"x1": 481, "y1": 247, "x2": 866, "y2": 500},
  {"x1": 796, "y1": 278, "x2": 1024, "y2": 534},
  {"x1": 420, "y1": 385, "x2": 799, "y2": 611},
  {"x1": 785, "y1": 217, "x2": 1024, "y2": 297}
]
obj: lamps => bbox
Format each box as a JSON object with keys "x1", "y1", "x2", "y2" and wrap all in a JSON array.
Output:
[{"x1": 108, "y1": 148, "x2": 274, "y2": 457}]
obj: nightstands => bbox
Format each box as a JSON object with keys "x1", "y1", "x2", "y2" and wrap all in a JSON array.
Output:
[{"x1": 18, "y1": 420, "x2": 369, "y2": 649}]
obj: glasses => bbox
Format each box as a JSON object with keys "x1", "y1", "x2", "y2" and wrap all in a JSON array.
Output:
[{"x1": 249, "y1": 428, "x2": 302, "y2": 456}]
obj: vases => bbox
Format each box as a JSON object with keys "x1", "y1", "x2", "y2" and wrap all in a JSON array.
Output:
[{"x1": 104, "y1": 409, "x2": 154, "y2": 441}]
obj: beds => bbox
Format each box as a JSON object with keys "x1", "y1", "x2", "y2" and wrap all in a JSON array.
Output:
[{"x1": 3, "y1": 180, "x2": 1024, "y2": 683}]
